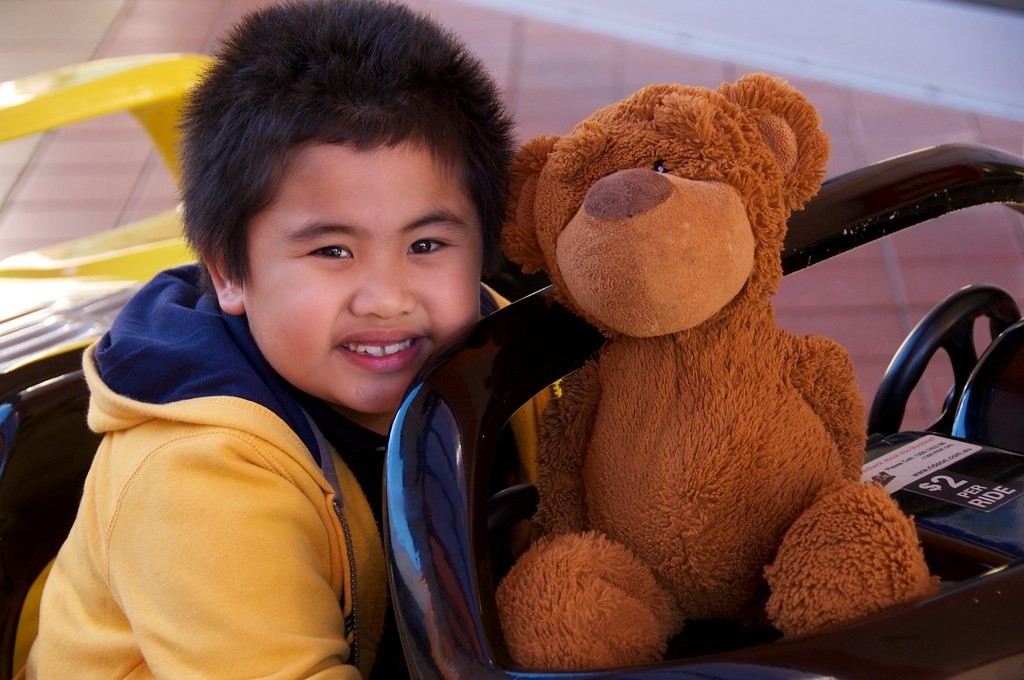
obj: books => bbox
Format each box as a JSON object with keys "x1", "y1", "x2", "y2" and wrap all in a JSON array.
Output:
[{"x1": 862, "y1": 431, "x2": 1024, "y2": 557}]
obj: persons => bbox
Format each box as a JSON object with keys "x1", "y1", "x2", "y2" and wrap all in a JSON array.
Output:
[{"x1": 11, "y1": 0, "x2": 517, "y2": 680}]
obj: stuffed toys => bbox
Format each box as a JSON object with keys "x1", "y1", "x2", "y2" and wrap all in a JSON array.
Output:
[{"x1": 498, "y1": 72, "x2": 936, "y2": 672}]
